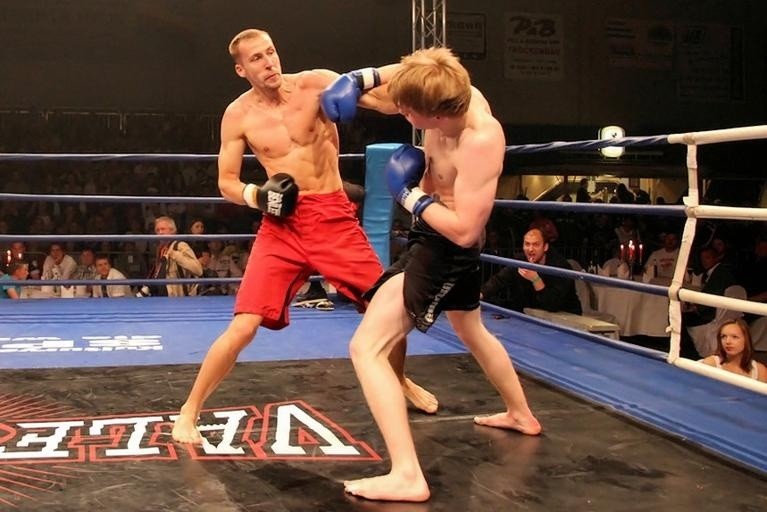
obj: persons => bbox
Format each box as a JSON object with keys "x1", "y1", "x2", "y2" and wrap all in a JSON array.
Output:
[
  {"x1": 169, "y1": 27, "x2": 439, "y2": 441},
  {"x1": 318, "y1": 46, "x2": 542, "y2": 501}
]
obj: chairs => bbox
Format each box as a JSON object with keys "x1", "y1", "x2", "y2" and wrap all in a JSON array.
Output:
[{"x1": 522, "y1": 226, "x2": 766, "y2": 367}]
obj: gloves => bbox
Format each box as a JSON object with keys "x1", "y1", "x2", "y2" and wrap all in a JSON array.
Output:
[
  {"x1": 319, "y1": 66, "x2": 382, "y2": 125},
  {"x1": 383, "y1": 143, "x2": 435, "y2": 220},
  {"x1": 241, "y1": 172, "x2": 300, "y2": 219}
]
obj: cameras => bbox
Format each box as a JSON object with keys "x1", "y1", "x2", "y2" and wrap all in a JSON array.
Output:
[{"x1": 136, "y1": 280, "x2": 158, "y2": 298}]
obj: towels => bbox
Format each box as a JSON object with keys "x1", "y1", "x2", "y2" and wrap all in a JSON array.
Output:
[{"x1": 58, "y1": 284, "x2": 74, "y2": 297}]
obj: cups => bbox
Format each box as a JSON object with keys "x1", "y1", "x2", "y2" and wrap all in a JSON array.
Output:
[
  {"x1": 40, "y1": 285, "x2": 54, "y2": 293},
  {"x1": 76, "y1": 286, "x2": 86, "y2": 295}
]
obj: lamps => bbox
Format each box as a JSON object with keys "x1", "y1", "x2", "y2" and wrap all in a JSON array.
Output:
[{"x1": 597, "y1": 125, "x2": 625, "y2": 162}]
